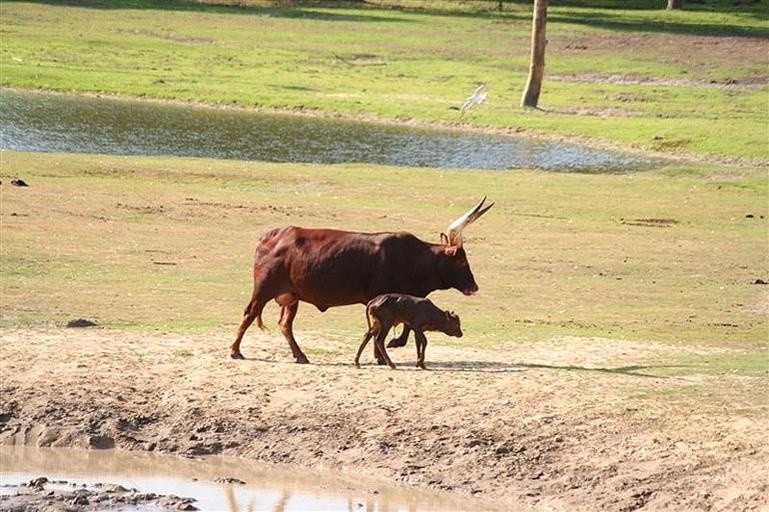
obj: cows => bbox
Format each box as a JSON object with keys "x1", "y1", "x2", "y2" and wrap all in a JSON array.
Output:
[
  {"x1": 364, "y1": 293, "x2": 464, "y2": 370},
  {"x1": 230, "y1": 195, "x2": 496, "y2": 364}
]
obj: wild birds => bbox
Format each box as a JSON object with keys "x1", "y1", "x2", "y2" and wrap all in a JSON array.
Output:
[{"x1": 459, "y1": 84, "x2": 489, "y2": 120}]
